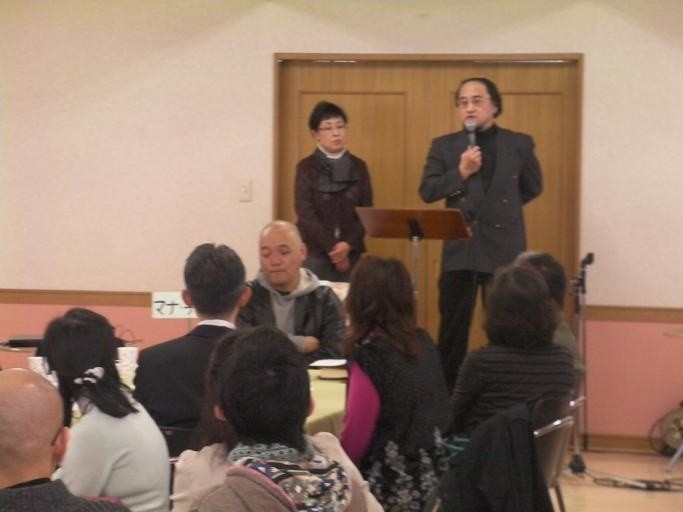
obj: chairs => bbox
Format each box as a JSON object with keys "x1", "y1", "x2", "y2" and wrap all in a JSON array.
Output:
[
  {"x1": 158, "y1": 425, "x2": 202, "y2": 507},
  {"x1": 534, "y1": 392, "x2": 575, "y2": 512},
  {"x1": 566, "y1": 379, "x2": 586, "y2": 472}
]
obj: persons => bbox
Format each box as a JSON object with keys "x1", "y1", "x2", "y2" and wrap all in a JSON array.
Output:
[
  {"x1": 131, "y1": 242, "x2": 253, "y2": 459},
  {"x1": 293, "y1": 100, "x2": 374, "y2": 283},
  {"x1": 338, "y1": 253, "x2": 453, "y2": 512},
  {"x1": 450, "y1": 260, "x2": 576, "y2": 433},
  {"x1": 417, "y1": 76, "x2": 544, "y2": 391},
  {"x1": 0, "y1": 366, "x2": 132, "y2": 512},
  {"x1": 233, "y1": 219, "x2": 347, "y2": 370},
  {"x1": 512, "y1": 247, "x2": 587, "y2": 396},
  {"x1": 35, "y1": 306, "x2": 172, "y2": 512}
]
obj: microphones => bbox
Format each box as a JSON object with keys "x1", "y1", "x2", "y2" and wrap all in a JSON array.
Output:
[{"x1": 464, "y1": 118, "x2": 478, "y2": 147}]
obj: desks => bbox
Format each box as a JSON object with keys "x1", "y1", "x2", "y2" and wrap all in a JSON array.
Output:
[{"x1": 40, "y1": 367, "x2": 347, "y2": 481}]
